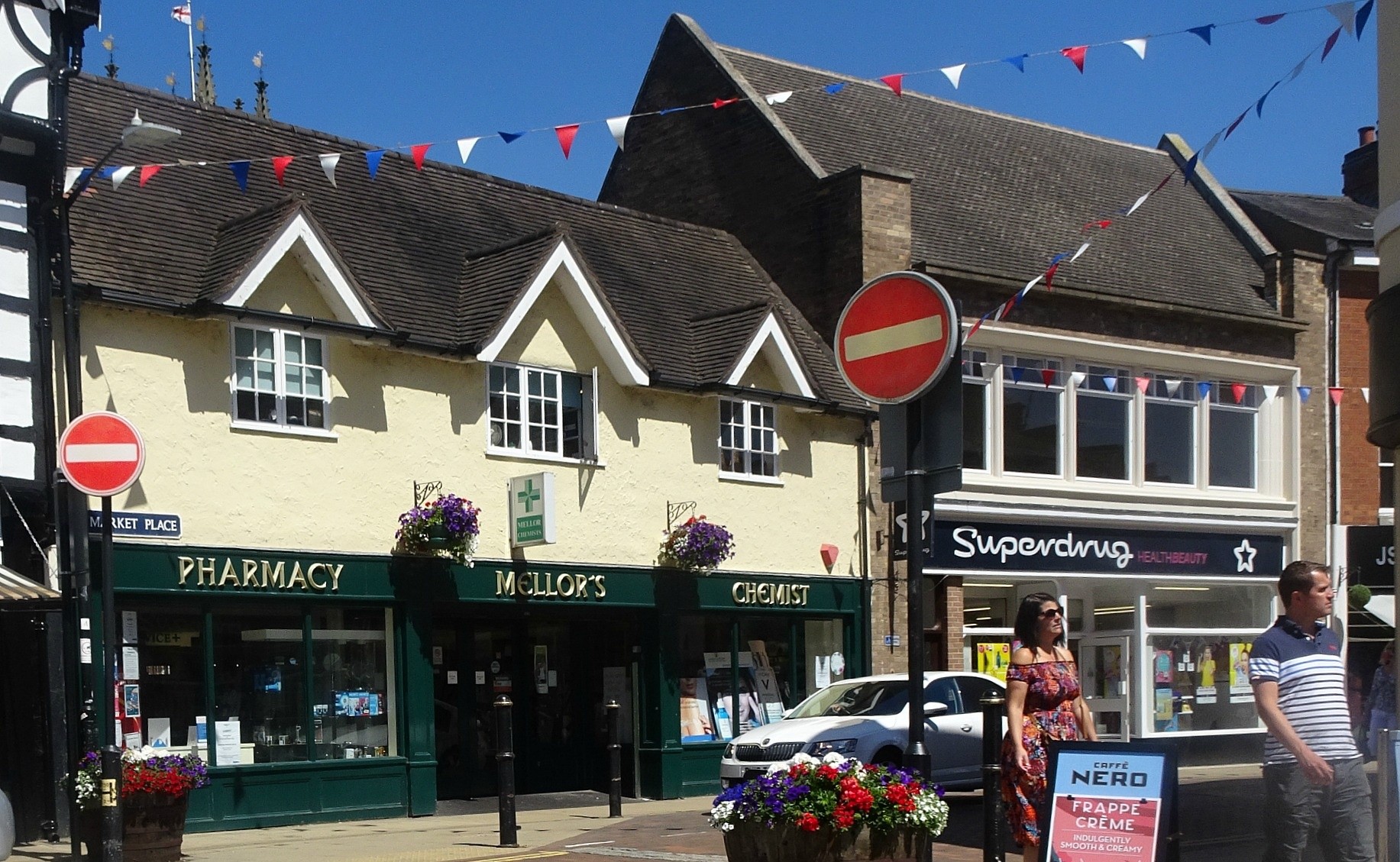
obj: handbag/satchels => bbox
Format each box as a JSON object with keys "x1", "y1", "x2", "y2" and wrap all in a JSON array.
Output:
[{"x1": 1356, "y1": 723, "x2": 1371, "y2": 764}]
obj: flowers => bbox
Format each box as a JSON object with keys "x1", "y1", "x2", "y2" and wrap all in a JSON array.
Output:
[
  {"x1": 395, "y1": 492, "x2": 483, "y2": 569},
  {"x1": 72, "y1": 747, "x2": 213, "y2": 813},
  {"x1": 700, "y1": 749, "x2": 950, "y2": 840},
  {"x1": 656, "y1": 514, "x2": 736, "y2": 577}
]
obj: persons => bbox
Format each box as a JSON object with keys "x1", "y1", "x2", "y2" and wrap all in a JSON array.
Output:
[
  {"x1": 1357, "y1": 648, "x2": 1398, "y2": 762},
  {"x1": 1197, "y1": 646, "x2": 1216, "y2": 689},
  {"x1": 679, "y1": 677, "x2": 712, "y2": 741},
  {"x1": 1248, "y1": 559, "x2": 1382, "y2": 862},
  {"x1": 1233, "y1": 643, "x2": 1250, "y2": 687},
  {"x1": 1002, "y1": 591, "x2": 1099, "y2": 862},
  {"x1": 715, "y1": 669, "x2": 763, "y2": 741}
]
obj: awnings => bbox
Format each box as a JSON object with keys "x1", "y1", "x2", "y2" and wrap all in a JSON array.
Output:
[{"x1": 0, "y1": 563, "x2": 67, "y2": 614}]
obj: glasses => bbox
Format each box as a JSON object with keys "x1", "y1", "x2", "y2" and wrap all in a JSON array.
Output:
[{"x1": 1037, "y1": 608, "x2": 1064, "y2": 617}]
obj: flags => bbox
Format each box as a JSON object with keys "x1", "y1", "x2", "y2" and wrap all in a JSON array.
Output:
[{"x1": 170, "y1": 6, "x2": 192, "y2": 24}]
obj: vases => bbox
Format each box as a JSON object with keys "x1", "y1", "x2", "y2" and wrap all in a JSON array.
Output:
[
  {"x1": 680, "y1": 545, "x2": 714, "y2": 568},
  {"x1": 722, "y1": 819, "x2": 932, "y2": 862},
  {"x1": 415, "y1": 525, "x2": 453, "y2": 550},
  {"x1": 82, "y1": 790, "x2": 190, "y2": 862}
]
